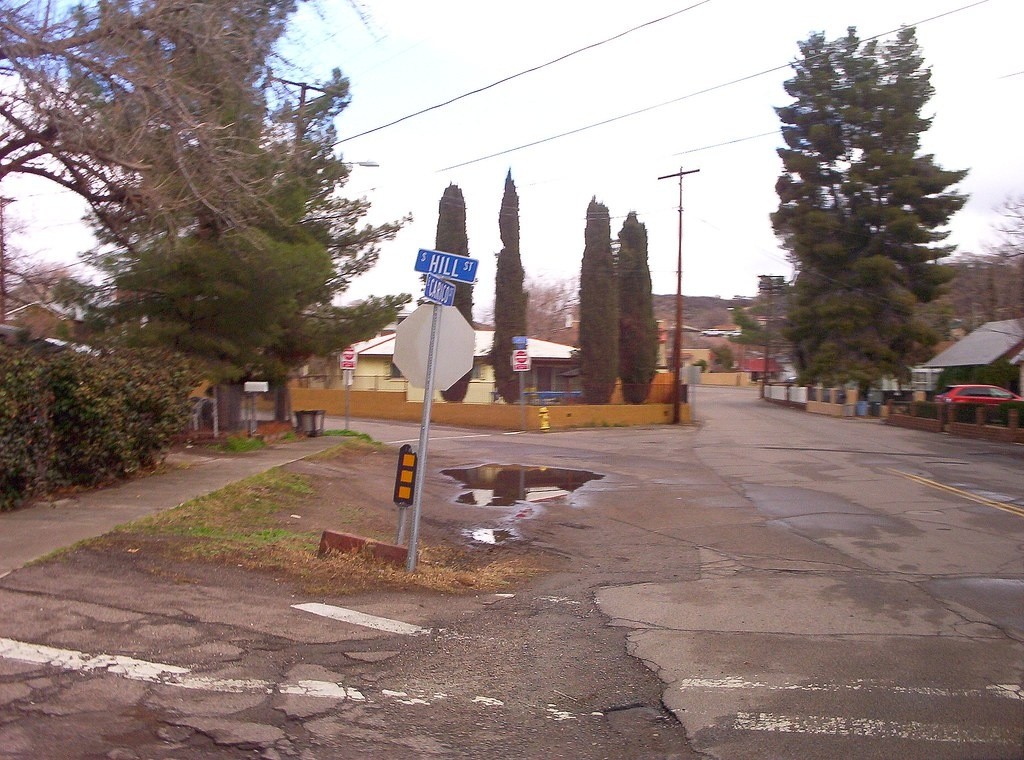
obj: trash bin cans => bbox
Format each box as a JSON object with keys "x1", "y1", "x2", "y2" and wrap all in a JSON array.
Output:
[
  {"x1": 871, "y1": 401, "x2": 881, "y2": 416},
  {"x1": 856, "y1": 401, "x2": 868, "y2": 416},
  {"x1": 294, "y1": 409, "x2": 327, "y2": 438}
]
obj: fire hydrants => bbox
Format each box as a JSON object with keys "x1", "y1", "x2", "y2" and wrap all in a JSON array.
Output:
[{"x1": 538, "y1": 407, "x2": 551, "y2": 430}]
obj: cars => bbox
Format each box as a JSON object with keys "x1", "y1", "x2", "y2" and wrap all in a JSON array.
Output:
[
  {"x1": 933, "y1": 385, "x2": 1023, "y2": 404},
  {"x1": 784, "y1": 376, "x2": 797, "y2": 384}
]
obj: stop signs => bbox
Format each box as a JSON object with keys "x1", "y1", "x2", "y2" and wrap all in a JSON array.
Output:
[
  {"x1": 343, "y1": 347, "x2": 354, "y2": 359},
  {"x1": 516, "y1": 352, "x2": 526, "y2": 363}
]
